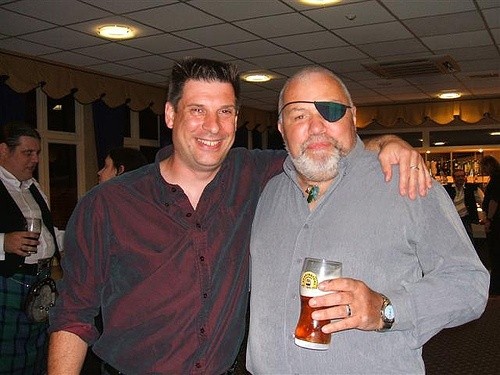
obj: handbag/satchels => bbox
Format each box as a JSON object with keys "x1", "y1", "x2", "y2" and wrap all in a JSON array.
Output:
[{"x1": 23, "y1": 274, "x2": 58, "y2": 323}]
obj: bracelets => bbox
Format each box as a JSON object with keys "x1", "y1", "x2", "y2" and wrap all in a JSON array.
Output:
[{"x1": 487, "y1": 218, "x2": 493, "y2": 221}]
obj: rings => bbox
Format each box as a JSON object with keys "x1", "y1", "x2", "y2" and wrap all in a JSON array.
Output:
[
  {"x1": 345, "y1": 304, "x2": 351, "y2": 318},
  {"x1": 410, "y1": 165, "x2": 419, "y2": 170}
]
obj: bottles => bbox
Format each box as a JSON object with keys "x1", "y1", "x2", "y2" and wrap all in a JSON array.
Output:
[{"x1": 425, "y1": 158, "x2": 482, "y2": 177}]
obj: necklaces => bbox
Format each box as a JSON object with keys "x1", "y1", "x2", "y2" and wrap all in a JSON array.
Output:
[{"x1": 296, "y1": 172, "x2": 321, "y2": 204}]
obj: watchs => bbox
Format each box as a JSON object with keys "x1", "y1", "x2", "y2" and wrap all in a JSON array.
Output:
[{"x1": 375, "y1": 293, "x2": 395, "y2": 333}]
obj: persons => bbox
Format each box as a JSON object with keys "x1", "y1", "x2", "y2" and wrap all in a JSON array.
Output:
[
  {"x1": 97, "y1": 148, "x2": 144, "y2": 181},
  {"x1": 243, "y1": 67, "x2": 492, "y2": 375},
  {"x1": 0, "y1": 122, "x2": 66, "y2": 375},
  {"x1": 442, "y1": 169, "x2": 479, "y2": 243},
  {"x1": 49, "y1": 56, "x2": 432, "y2": 375},
  {"x1": 481, "y1": 156, "x2": 500, "y2": 294}
]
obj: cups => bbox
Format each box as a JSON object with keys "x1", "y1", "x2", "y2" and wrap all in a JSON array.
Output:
[
  {"x1": 294, "y1": 257, "x2": 342, "y2": 351},
  {"x1": 23, "y1": 216, "x2": 42, "y2": 254}
]
